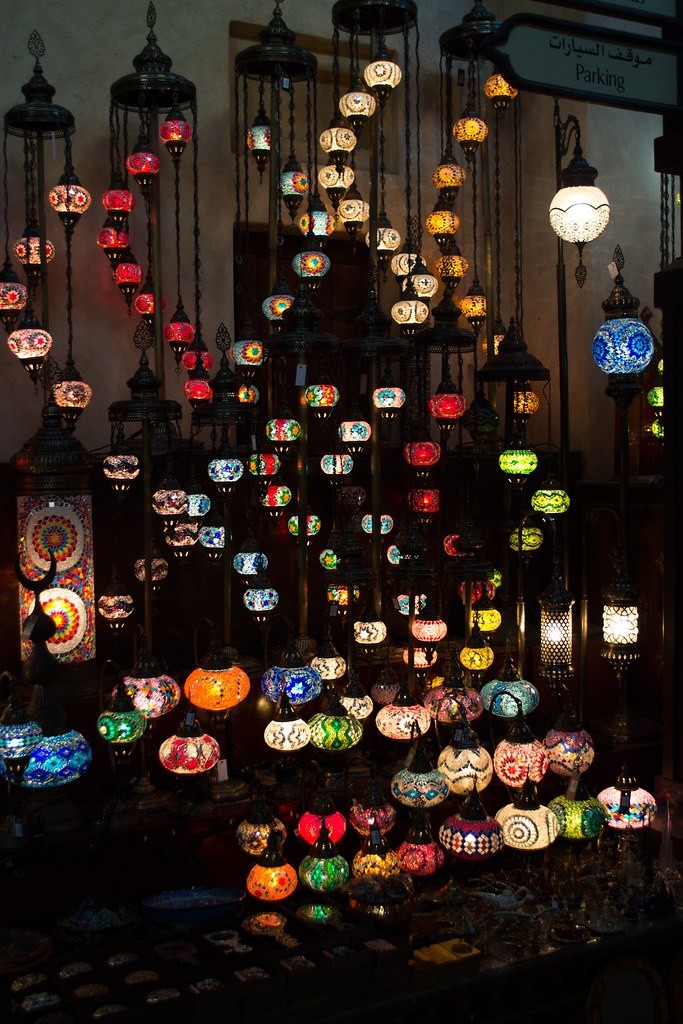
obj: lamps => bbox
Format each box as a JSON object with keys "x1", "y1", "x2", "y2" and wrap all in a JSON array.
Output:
[{"x1": 0, "y1": 0, "x2": 661, "y2": 904}]
